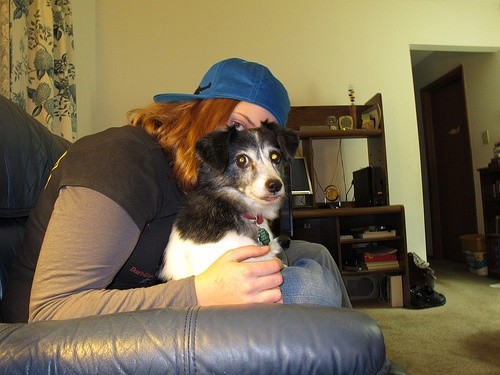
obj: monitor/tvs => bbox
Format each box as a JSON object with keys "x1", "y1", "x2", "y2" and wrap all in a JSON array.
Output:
[{"x1": 290, "y1": 156, "x2": 313, "y2": 195}]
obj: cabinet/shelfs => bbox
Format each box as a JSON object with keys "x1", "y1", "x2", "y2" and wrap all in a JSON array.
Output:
[
  {"x1": 287, "y1": 94, "x2": 410, "y2": 308},
  {"x1": 477, "y1": 166, "x2": 500, "y2": 279}
]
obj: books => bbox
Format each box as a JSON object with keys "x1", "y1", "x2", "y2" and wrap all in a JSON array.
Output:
[
  {"x1": 358, "y1": 231, "x2": 396, "y2": 238},
  {"x1": 357, "y1": 249, "x2": 399, "y2": 270}
]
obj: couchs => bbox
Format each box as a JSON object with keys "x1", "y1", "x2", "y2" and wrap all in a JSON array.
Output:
[{"x1": 0, "y1": 95, "x2": 406, "y2": 375}]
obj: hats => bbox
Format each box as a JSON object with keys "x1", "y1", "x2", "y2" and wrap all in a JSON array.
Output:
[{"x1": 154, "y1": 57, "x2": 290, "y2": 130}]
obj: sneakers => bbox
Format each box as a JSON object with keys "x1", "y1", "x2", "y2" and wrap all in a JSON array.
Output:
[{"x1": 409, "y1": 285, "x2": 446, "y2": 309}]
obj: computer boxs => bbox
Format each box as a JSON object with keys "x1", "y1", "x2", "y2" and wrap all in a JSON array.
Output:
[{"x1": 352, "y1": 166, "x2": 388, "y2": 207}]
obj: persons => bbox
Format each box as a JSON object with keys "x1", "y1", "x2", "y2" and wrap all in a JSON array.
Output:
[{"x1": 0, "y1": 58, "x2": 353, "y2": 323}]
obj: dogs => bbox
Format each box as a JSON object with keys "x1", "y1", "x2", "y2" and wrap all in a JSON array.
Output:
[{"x1": 128, "y1": 118, "x2": 301, "y2": 290}]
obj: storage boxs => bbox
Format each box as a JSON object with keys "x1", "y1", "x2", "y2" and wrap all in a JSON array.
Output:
[{"x1": 460, "y1": 233, "x2": 486, "y2": 252}]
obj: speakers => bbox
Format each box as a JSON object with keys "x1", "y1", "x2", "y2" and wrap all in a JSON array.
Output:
[{"x1": 339, "y1": 115, "x2": 354, "y2": 130}]
